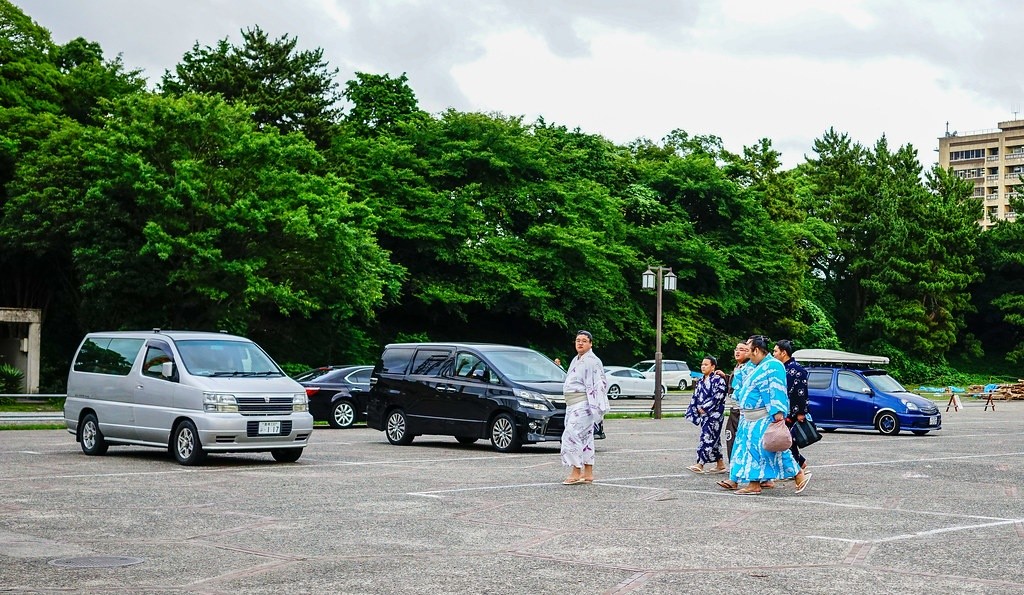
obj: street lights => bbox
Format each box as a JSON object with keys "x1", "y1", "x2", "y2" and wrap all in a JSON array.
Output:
[{"x1": 641, "y1": 264, "x2": 677, "y2": 420}]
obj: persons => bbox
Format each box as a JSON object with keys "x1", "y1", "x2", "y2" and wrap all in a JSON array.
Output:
[
  {"x1": 551, "y1": 358, "x2": 565, "y2": 380},
  {"x1": 561, "y1": 330, "x2": 609, "y2": 484},
  {"x1": 715, "y1": 334, "x2": 823, "y2": 496},
  {"x1": 684, "y1": 353, "x2": 728, "y2": 475}
]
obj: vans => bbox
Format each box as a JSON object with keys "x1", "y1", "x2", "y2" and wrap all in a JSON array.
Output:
[
  {"x1": 64, "y1": 328, "x2": 314, "y2": 467},
  {"x1": 367, "y1": 342, "x2": 606, "y2": 454},
  {"x1": 630, "y1": 359, "x2": 692, "y2": 391}
]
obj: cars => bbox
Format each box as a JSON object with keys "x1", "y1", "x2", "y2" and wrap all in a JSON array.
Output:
[
  {"x1": 291, "y1": 364, "x2": 375, "y2": 429},
  {"x1": 603, "y1": 366, "x2": 667, "y2": 401},
  {"x1": 791, "y1": 349, "x2": 942, "y2": 436}
]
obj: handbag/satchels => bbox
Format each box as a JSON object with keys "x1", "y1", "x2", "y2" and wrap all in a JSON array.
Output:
[{"x1": 792, "y1": 417, "x2": 823, "y2": 448}]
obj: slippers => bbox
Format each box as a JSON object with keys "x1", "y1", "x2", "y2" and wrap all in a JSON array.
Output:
[
  {"x1": 580, "y1": 480, "x2": 591, "y2": 483},
  {"x1": 717, "y1": 481, "x2": 737, "y2": 490},
  {"x1": 796, "y1": 474, "x2": 812, "y2": 493},
  {"x1": 734, "y1": 488, "x2": 761, "y2": 494},
  {"x1": 710, "y1": 468, "x2": 727, "y2": 473},
  {"x1": 762, "y1": 485, "x2": 773, "y2": 488},
  {"x1": 687, "y1": 466, "x2": 706, "y2": 473},
  {"x1": 563, "y1": 478, "x2": 585, "y2": 484}
]
